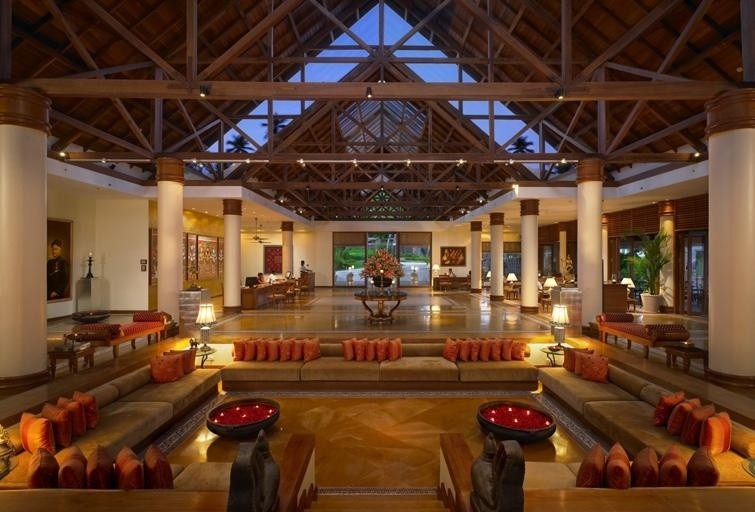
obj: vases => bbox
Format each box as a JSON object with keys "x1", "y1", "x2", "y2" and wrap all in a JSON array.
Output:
[{"x1": 372, "y1": 276, "x2": 393, "y2": 288}]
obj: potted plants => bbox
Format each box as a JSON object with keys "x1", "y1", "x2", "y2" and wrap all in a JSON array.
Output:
[{"x1": 620, "y1": 225, "x2": 673, "y2": 313}]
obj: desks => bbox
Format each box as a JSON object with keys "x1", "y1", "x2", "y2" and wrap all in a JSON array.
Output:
[
  {"x1": 353, "y1": 288, "x2": 408, "y2": 323},
  {"x1": 540, "y1": 346, "x2": 571, "y2": 368},
  {"x1": 77, "y1": 278, "x2": 112, "y2": 312},
  {"x1": 188, "y1": 347, "x2": 217, "y2": 368},
  {"x1": 240, "y1": 282, "x2": 288, "y2": 310},
  {"x1": 433, "y1": 276, "x2": 472, "y2": 292},
  {"x1": 46, "y1": 344, "x2": 98, "y2": 379},
  {"x1": 604, "y1": 281, "x2": 628, "y2": 313},
  {"x1": 662, "y1": 344, "x2": 709, "y2": 375},
  {"x1": 626, "y1": 297, "x2": 637, "y2": 313}
]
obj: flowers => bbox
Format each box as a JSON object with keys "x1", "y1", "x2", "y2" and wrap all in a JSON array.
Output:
[{"x1": 358, "y1": 247, "x2": 406, "y2": 281}]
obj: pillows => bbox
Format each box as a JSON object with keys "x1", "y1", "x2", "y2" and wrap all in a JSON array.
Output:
[
  {"x1": 17, "y1": 389, "x2": 99, "y2": 458},
  {"x1": 652, "y1": 388, "x2": 733, "y2": 457},
  {"x1": 26, "y1": 441, "x2": 175, "y2": 491},
  {"x1": 340, "y1": 336, "x2": 403, "y2": 364},
  {"x1": 561, "y1": 346, "x2": 610, "y2": 386},
  {"x1": 232, "y1": 336, "x2": 323, "y2": 364},
  {"x1": 149, "y1": 345, "x2": 199, "y2": 384},
  {"x1": 575, "y1": 440, "x2": 721, "y2": 489},
  {"x1": 440, "y1": 336, "x2": 528, "y2": 363}
]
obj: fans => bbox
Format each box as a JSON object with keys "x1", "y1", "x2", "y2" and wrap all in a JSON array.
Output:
[
  {"x1": 249, "y1": 223, "x2": 271, "y2": 244},
  {"x1": 244, "y1": 217, "x2": 271, "y2": 242}
]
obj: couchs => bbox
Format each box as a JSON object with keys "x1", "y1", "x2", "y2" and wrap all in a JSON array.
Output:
[
  {"x1": 24, "y1": 440, "x2": 241, "y2": 491},
  {"x1": 537, "y1": 345, "x2": 755, "y2": 483},
  {"x1": 219, "y1": 336, "x2": 541, "y2": 392},
  {"x1": 511, "y1": 441, "x2": 723, "y2": 488},
  {"x1": 0, "y1": 349, "x2": 222, "y2": 478}
]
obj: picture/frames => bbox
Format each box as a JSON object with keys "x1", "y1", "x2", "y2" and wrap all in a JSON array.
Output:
[
  {"x1": 440, "y1": 245, "x2": 466, "y2": 268},
  {"x1": 44, "y1": 216, "x2": 74, "y2": 305},
  {"x1": 262, "y1": 244, "x2": 284, "y2": 276}
]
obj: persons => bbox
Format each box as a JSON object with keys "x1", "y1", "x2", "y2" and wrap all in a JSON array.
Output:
[
  {"x1": 269, "y1": 270, "x2": 277, "y2": 283},
  {"x1": 448, "y1": 268, "x2": 454, "y2": 277},
  {"x1": 299, "y1": 259, "x2": 308, "y2": 281},
  {"x1": 256, "y1": 272, "x2": 266, "y2": 283},
  {"x1": 47, "y1": 238, "x2": 70, "y2": 299}
]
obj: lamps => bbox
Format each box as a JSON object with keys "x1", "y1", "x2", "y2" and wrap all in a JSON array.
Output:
[
  {"x1": 363, "y1": 87, "x2": 374, "y2": 104},
  {"x1": 200, "y1": 82, "x2": 212, "y2": 100},
  {"x1": 432, "y1": 263, "x2": 440, "y2": 277},
  {"x1": 193, "y1": 303, "x2": 218, "y2": 352},
  {"x1": 551, "y1": 304, "x2": 571, "y2": 343},
  {"x1": 83, "y1": 252, "x2": 95, "y2": 279},
  {"x1": 554, "y1": 87, "x2": 567, "y2": 98},
  {"x1": 620, "y1": 277, "x2": 637, "y2": 299},
  {"x1": 485, "y1": 270, "x2": 559, "y2": 311}
]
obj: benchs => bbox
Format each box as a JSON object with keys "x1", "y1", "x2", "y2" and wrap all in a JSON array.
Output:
[
  {"x1": 596, "y1": 311, "x2": 691, "y2": 359},
  {"x1": 64, "y1": 311, "x2": 167, "y2": 361}
]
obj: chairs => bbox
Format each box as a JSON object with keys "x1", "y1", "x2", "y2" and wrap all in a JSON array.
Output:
[
  {"x1": 263, "y1": 276, "x2": 312, "y2": 309},
  {"x1": 437, "y1": 273, "x2": 473, "y2": 293}
]
